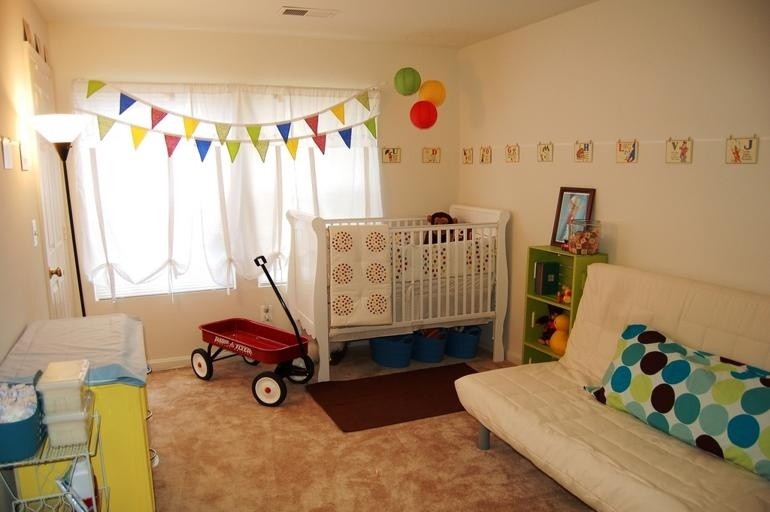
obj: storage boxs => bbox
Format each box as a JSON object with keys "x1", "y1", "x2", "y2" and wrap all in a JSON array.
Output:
[
  {"x1": 36, "y1": 359, "x2": 91, "y2": 410},
  {"x1": 43, "y1": 391, "x2": 95, "y2": 447}
]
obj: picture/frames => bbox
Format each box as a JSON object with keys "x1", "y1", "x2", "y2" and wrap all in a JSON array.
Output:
[{"x1": 548, "y1": 186, "x2": 595, "y2": 247}]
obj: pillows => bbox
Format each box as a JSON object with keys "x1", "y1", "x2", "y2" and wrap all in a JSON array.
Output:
[{"x1": 579, "y1": 320, "x2": 769, "y2": 482}]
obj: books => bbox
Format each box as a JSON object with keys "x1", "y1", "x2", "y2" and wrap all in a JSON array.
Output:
[{"x1": 534, "y1": 260, "x2": 560, "y2": 296}]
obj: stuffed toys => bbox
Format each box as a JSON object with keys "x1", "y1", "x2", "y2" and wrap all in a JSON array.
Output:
[
  {"x1": 562, "y1": 289, "x2": 572, "y2": 304},
  {"x1": 460, "y1": 228, "x2": 472, "y2": 241},
  {"x1": 548, "y1": 314, "x2": 570, "y2": 356},
  {"x1": 423, "y1": 212, "x2": 458, "y2": 243},
  {"x1": 535, "y1": 303, "x2": 564, "y2": 345}
]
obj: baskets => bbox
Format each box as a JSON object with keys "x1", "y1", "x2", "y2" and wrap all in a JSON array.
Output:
[
  {"x1": 368, "y1": 333, "x2": 412, "y2": 369},
  {"x1": 448, "y1": 326, "x2": 483, "y2": 360},
  {"x1": 0, "y1": 369, "x2": 47, "y2": 464},
  {"x1": 412, "y1": 327, "x2": 449, "y2": 363}
]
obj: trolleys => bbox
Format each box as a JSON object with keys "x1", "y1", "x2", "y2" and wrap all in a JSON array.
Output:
[{"x1": 189, "y1": 254, "x2": 314, "y2": 407}]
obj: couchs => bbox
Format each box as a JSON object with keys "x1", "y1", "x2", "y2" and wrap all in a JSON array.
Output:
[{"x1": 453, "y1": 260, "x2": 770, "y2": 510}]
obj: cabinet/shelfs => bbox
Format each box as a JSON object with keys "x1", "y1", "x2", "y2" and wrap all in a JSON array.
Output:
[
  {"x1": 522, "y1": 244, "x2": 609, "y2": 364},
  {"x1": 1, "y1": 411, "x2": 111, "y2": 510},
  {"x1": 2, "y1": 315, "x2": 158, "y2": 511}
]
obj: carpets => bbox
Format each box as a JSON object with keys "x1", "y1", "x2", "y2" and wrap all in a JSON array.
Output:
[{"x1": 304, "y1": 361, "x2": 479, "y2": 432}]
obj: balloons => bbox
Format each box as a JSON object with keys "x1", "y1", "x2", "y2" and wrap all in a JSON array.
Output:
[{"x1": 393, "y1": 67, "x2": 447, "y2": 130}]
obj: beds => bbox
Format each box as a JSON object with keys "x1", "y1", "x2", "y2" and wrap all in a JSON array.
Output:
[{"x1": 282, "y1": 204, "x2": 512, "y2": 384}]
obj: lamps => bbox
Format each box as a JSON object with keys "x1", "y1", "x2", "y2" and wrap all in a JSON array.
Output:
[{"x1": 35, "y1": 111, "x2": 91, "y2": 315}]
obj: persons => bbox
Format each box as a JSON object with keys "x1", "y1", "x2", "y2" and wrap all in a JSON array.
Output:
[{"x1": 560, "y1": 192, "x2": 582, "y2": 243}]
